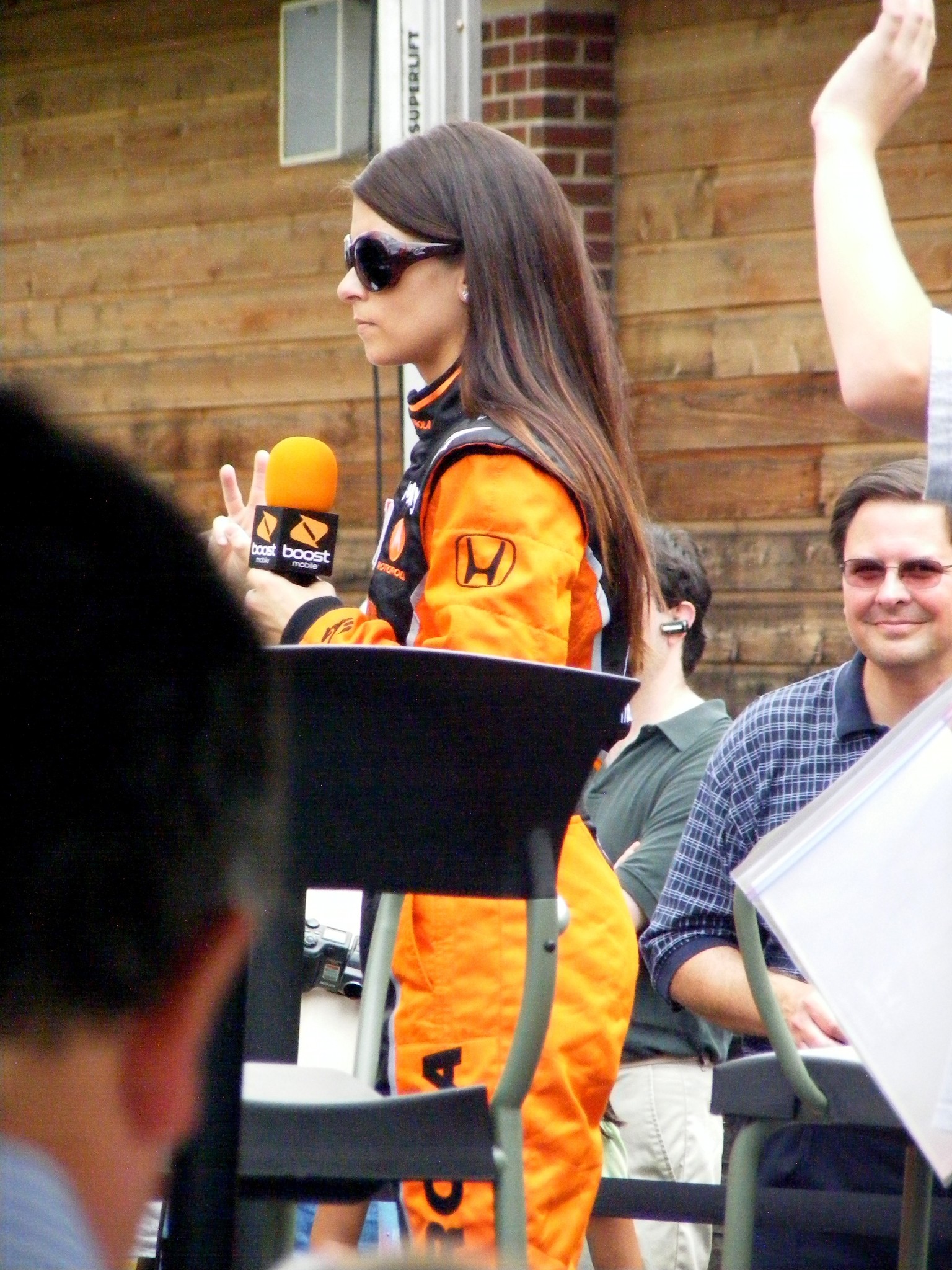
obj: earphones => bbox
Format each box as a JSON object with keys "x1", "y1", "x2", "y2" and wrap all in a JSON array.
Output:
[{"x1": 659, "y1": 620, "x2": 688, "y2": 634}]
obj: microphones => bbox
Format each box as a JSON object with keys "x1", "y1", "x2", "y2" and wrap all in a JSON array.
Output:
[{"x1": 247, "y1": 436, "x2": 339, "y2": 588}]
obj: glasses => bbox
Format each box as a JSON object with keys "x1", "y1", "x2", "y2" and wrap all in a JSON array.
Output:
[
  {"x1": 343, "y1": 230, "x2": 461, "y2": 291},
  {"x1": 838, "y1": 559, "x2": 952, "y2": 590}
]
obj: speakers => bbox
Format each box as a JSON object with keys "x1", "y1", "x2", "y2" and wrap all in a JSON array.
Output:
[{"x1": 279, "y1": 1, "x2": 375, "y2": 167}]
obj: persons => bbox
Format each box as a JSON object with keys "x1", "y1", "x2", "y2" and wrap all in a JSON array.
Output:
[
  {"x1": 0, "y1": 364, "x2": 295, "y2": 1269},
  {"x1": 552, "y1": 513, "x2": 753, "y2": 1267},
  {"x1": 121, "y1": 819, "x2": 394, "y2": 1269},
  {"x1": 631, "y1": 459, "x2": 952, "y2": 1269},
  {"x1": 785, "y1": 0, "x2": 952, "y2": 523},
  {"x1": 193, "y1": 108, "x2": 652, "y2": 1267}
]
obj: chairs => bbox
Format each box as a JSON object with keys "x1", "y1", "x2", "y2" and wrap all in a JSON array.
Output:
[{"x1": 240, "y1": 641, "x2": 933, "y2": 1270}]
